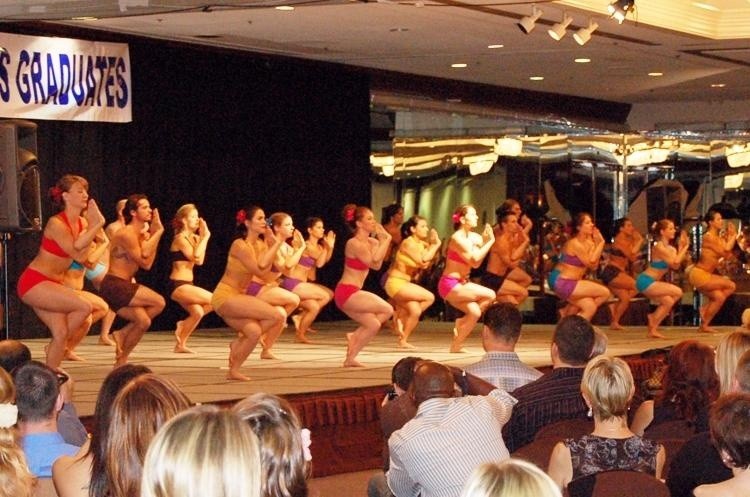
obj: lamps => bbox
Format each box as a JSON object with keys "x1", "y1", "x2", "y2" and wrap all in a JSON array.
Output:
[
  {"x1": 606, "y1": 0, "x2": 642, "y2": 27},
  {"x1": 513, "y1": 4, "x2": 605, "y2": 50}
]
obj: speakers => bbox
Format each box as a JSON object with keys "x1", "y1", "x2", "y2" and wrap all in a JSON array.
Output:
[{"x1": 0, "y1": 119, "x2": 43, "y2": 233}]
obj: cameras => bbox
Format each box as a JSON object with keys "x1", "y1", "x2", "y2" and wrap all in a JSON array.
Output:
[{"x1": 385, "y1": 388, "x2": 399, "y2": 402}]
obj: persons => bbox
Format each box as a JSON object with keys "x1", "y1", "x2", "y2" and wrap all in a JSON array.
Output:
[
  {"x1": 367, "y1": 302, "x2": 750, "y2": 496},
  {"x1": 17, "y1": 176, "x2": 750, "y2": 382},
  {"x1": 1, "y1": 340, "x2": 313, "y2": 496}
]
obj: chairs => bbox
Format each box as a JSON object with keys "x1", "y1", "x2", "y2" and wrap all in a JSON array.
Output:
[{"x1": 503, "y1": 403, "x2": 750, "y2": 497}]
